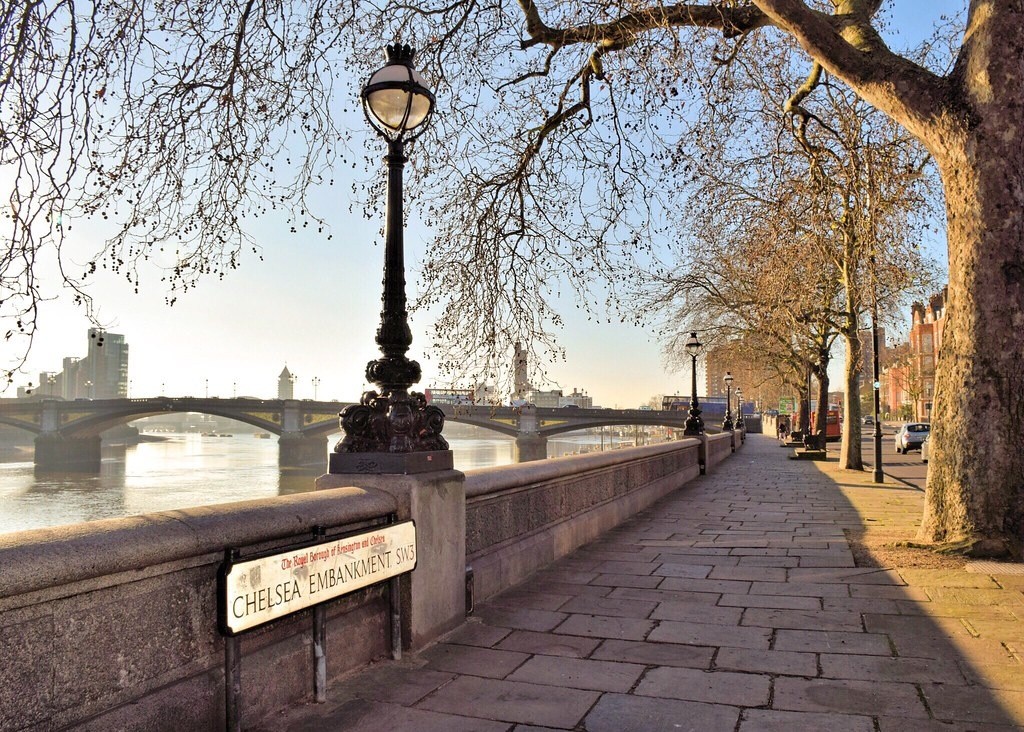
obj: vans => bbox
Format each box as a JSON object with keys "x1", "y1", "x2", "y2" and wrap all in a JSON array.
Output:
[
  {"x1": 521, "y1": 403, "x2": 537, "y2": 408},
  {"x1": 562, "y1": 404, "x2": 578, "y2": 408},
  {"x1": 74, "y1": 398, "x2": 93, "y2": 401}
]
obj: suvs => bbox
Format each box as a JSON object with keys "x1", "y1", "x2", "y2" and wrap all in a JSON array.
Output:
[{"x1": 895, "y1": 423, "x2": 931, "y2": 454}]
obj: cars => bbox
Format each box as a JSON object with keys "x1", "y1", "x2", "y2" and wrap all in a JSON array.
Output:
[
  {"x1": 864, "y1": 415, "x2": 875, "y2": 426},
  {"x1": 921, "y1": 434, "x2": 930, "y2": 462}
]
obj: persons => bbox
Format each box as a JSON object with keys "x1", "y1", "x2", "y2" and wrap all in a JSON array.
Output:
[{"x1": 779, "y1": 418, "x2": 790, "y2": 439}]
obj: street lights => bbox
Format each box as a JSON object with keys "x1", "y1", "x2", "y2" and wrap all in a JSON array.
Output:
[
  {"x1": 234, "y1": 383, "x2": 236, "y2": 398},
  {"x1": 683, "y1": 332, "x2": 705, "y2": 435},
  {"x1": 234, "y1": 383, "x2": 236, "y2": 399},
  {"x1": 205, "y1": 379, "x2": 208, "y2": 398},
  {"x1": 332, "y1": 46, "x2": 452, "y2": 470},
  {"x1": 362, "y1": 383, "x2": 365, "y2": 392},
  {"x1": 289, "y1": 373, "x2": 297, "y2": 400},
  {"x1": 734, "y1": 387, "x2": 745, "y2": 429},
  {"x1": 130, "y1": 380, "x2": 132, "y2": 399},
  {"x1": 162, "y1": 382, "x2": 165, "y2": 396},
  {"x1": 312, "y1": 377, "x2": 320, "y2": 401},
  {"x1": 47, "y1": 375, "x2": 56, "y2": 400},
  {"x1": 84, "y1": 380, "x2": 94, "y2": 398},
  {"x1": 723, "y1": 372, "x2": 733, "y2": 430}
]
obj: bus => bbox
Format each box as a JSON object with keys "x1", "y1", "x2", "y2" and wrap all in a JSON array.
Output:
[
  {"x1": 424, "y1": 388, "x2": 475, "y2": 406},
  {"x1": 661, "y1": 395, "x2": 754, "y2": 416},
  {"x1": 811, "y1": 400, "x2": 841, "y2": 441}
]
